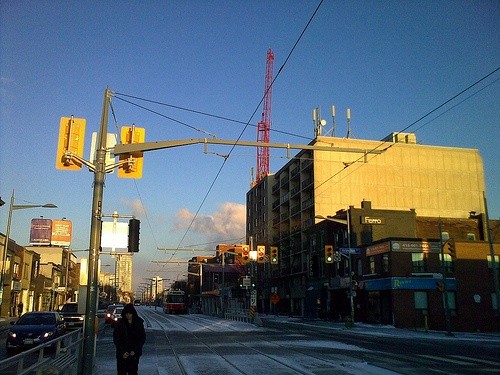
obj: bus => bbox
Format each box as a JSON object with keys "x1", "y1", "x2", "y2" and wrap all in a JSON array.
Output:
[{"x1": 162, "y1": 288, "x2": 188, "y2": 314}]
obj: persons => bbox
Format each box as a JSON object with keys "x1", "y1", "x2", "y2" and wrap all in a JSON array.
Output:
[
  {"x1": 112, "y1": 303, "x2": 146, "y2": 375},
  {"x1": 16, "y1": 301, "x2": 23, "y2": 318}
]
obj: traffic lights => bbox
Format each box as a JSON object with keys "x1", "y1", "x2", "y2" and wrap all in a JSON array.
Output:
[
  {"x1": 270, "y1": 246, "x2": 279, "y2": 265},
  {"x1": 250, "y1": 277, "x2": 256, "y2": 288},
  {"x1": 257, "y1": 245, "x2": 265, "y2": 263},
  {"x1": 241, "y1": 244, "x2": 250, "y2": 262},
  {"x1": 239, "y1": 277, "x2": 244, "y2": 288},
  {"x1": 436, "y1": 281, "x2": 444, "y2": 292},
  {"x1": 324, "y1": 245, "x2": 334, "y2": 263},
  {"x1": 447, "y1": 240, "x2": 457, "y2": 259},
  {"x1": 353, "y1": 280, "x2": 359, "y2": 290}
]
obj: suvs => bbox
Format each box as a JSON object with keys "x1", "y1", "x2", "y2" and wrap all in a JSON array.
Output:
[{"x1": 58, "y1": 303, "x2": 85, "y2": 330}]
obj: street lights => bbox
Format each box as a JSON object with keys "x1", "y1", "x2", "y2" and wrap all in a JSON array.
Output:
[
  {"x1": 315, "y1": 208, "x2": 355, "y2": 327},
  {"x1": 63, "y1": 246, "x2": 90, "y2": 303},
  {"x1": 0, "y1": 188, "x2": 59, "y2": 292}
]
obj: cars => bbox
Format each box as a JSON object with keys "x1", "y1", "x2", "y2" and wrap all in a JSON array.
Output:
[
  {"x1": 5, "y1": 311, "x2": 66, "y2": 353},
  {"x1": 109, "y1": 307, "x2": 124, "y2": 328},
  {"x1": 104, "y1": 304, "x2": 124, "y2": 324}
]
